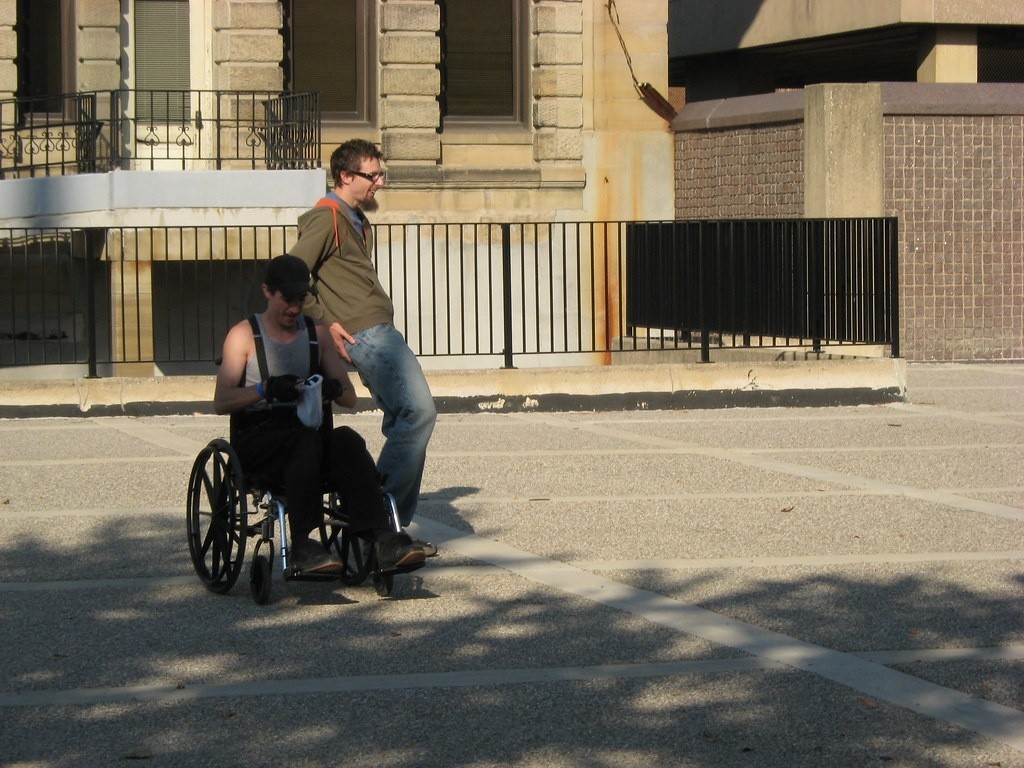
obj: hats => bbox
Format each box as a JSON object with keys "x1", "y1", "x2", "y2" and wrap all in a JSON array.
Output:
[{"x1": 265, "y1": 254, "x2": 310, "y2": 299}]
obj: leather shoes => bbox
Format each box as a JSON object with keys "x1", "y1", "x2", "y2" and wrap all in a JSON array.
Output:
[
  {"x1": 286, "y1": 540, "x2": 343, "y2": 574},
  {"x1": 373, "y1": 533, "x2": 425, "y2": 571}
]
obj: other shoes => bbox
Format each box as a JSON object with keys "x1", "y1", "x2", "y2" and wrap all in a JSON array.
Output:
[{"x1": 401, "y1": 532, "x2": 437, "y2": 556}]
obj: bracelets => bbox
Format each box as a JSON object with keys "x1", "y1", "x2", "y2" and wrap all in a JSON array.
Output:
[{"x1": 256, "y1": 382, "x2": 265, "y2": 396}]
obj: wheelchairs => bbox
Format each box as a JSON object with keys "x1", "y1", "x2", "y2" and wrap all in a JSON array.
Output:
[{"x1": 185, "y1": 360, "x2": 440, "y2": 606}]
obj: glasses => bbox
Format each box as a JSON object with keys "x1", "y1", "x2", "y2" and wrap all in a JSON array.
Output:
[{"x1": 347, "y1": 169, "x2": 387, "y2": 183}]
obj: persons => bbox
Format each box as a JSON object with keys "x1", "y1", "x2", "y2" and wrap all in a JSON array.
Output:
[
  {"x1": 286, "y1": 138, "x2": 438, "y2": 559},
  {"x1": 214, "y1": 256, "x2": 425, "y2": 582}
]
